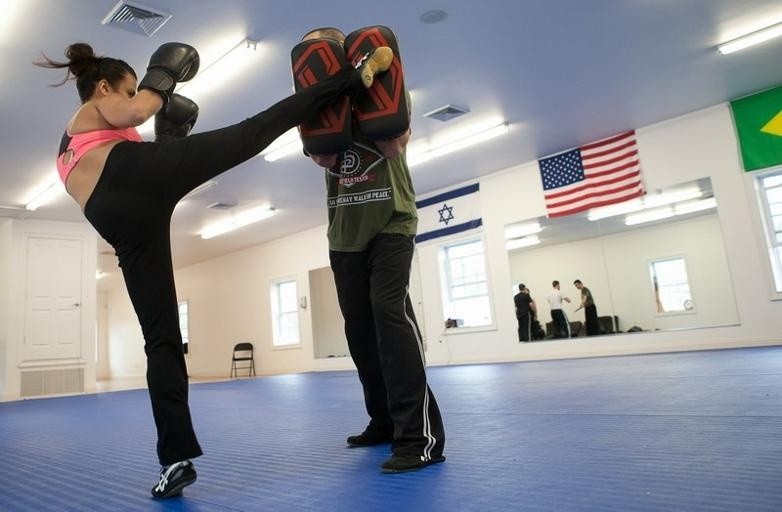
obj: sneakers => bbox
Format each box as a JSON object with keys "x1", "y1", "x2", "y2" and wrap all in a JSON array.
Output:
[
  {"x1": 346, "y1": 428, "x2": 387, "y2": 446},
  {"x1": 151, "y1": 460, "x2": 197, "y2": 499},
  {"x1": 361, "y1": 46, "x2": 393, "y2": 88},
  {"x1": 381, "y1": 453, "x2": 431, "y2": 473}
]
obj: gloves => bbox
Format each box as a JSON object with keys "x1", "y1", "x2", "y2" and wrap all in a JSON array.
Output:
[
  {"x1": 154, "y1": 93, "x2": 199, "y2": 141},
  {"x1": 138, "y1": 42, "x2": 200, "y2": 106}
]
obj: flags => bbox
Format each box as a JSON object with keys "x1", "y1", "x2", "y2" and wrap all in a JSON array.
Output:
[
  {"x1": 728, "y1": 85, "x2": 781, "y2": 175},
  {"x1": 537, "y1": 128, "x2": 647, "y2": 219},
  {"x1": 412, "y1": 181, "x2": 482, "y2": 244}
]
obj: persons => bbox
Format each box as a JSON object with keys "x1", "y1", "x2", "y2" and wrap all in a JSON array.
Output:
[
  {"x1": 572, "y1": 279, "x2": 598, "y2": 336},
  {"x1": 290, "y1": 27, "x2": 448, "y2": 470},
  {"x1": 515, "y1": 283, "x2": 534, "y2": 341},
  {"x1": 523, "y1": 287, "x2": 538, "y2": 340},
  {"x1": 548, "y1": 280, "x2": 572, "y2": 339},
  {"x1": 33, "y1": 42, "x2": 395, "y2": 497}
]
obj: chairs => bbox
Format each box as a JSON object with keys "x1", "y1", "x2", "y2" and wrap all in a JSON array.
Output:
[{"x1": 230, "y1": 343, "x2": 256, "y2": 379}]
